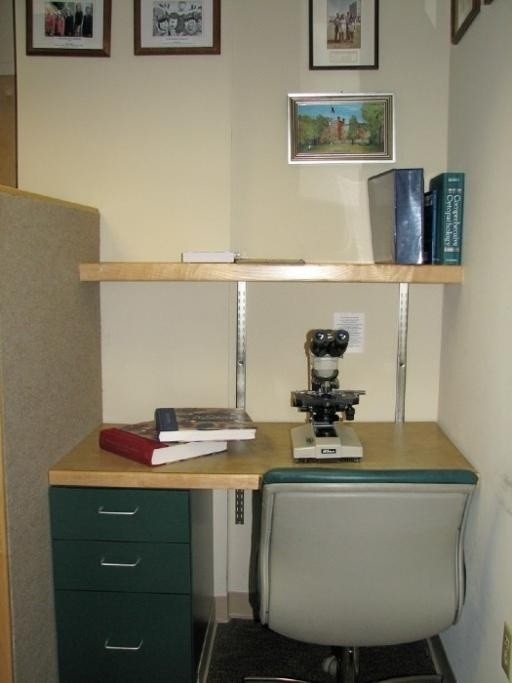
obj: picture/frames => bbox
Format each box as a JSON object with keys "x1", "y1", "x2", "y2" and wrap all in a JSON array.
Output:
[
  {"x1": 287, "y1": 93, "x2": 396, "y2": 164},
  {"x1": 309, "y1": 0, "x2": 379, "y2": 70},
  {"x1": 26, "y1": 0, "x2": 112, "y2": 57},
  {"x1": 450, "y1": 0, "x2": 480, "y2": 44},
  {"x1": 134, "y1": 0, "x2": 221, "y2": 55}
]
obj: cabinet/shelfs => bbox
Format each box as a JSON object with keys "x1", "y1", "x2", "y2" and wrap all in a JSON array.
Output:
[
  {"x1": 45, "y1": 261, "x2": 478, "y2": 683},
  {"x1": 49, "y1": 487, "x2": 196, "y2": 682}
]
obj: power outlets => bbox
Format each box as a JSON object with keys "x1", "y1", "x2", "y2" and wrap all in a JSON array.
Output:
[{"x1": 501, "y1": 622, "x2": 512, "y2": 682}]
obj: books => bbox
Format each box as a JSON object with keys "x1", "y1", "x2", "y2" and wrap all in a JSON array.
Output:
[
  {"x1": 98, "y1": 419, "x2": 229, "y2": 466},
  {"x1": 153, "y1": 406, "x2": 257, "y2": 443},
  {"x1": 368, "y1": 167, "x2": 425, "y2": 266},
  {"x1": 428, "y1": 171, "x2": 465, "y2": 265},
  {"x1": 180, "y1": 250, "x2": 236, "y2": 265},
  {"x1": 424, "y1": 189, "x2": 439, "y2": 264},
  {"x1": 233, "y1": 258, "x2": 305, "y2": 265}
]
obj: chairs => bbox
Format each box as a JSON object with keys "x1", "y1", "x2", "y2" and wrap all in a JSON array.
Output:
[{"x1": 235, "y1": 467, "x2": 477, "y2": 683}]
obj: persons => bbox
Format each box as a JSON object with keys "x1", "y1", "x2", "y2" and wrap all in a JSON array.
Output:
[
  {"x1": 333, "y1": 12, "x2": 357, "y2": 43},
  {"x1": 46, "y1": 1, "x2": 93, "y2": 38},
  {"x1": 153, "y1": 1, "x2": 202, "y2": 38},
  {"x1": 336, "y1": 116, "x2": 344, "y2": 140},
  {"x1": 339, "y1": 118, "x2": 345, "y2": 124}
]
